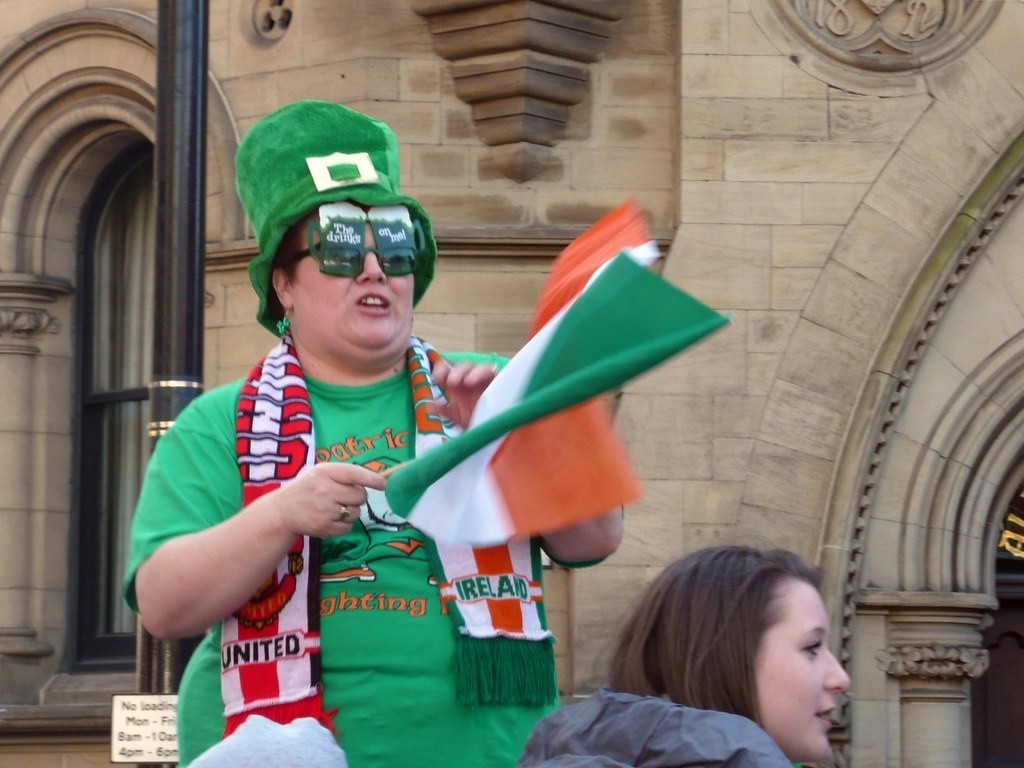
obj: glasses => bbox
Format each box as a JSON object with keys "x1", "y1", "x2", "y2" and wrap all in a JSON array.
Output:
[{"x1": 284, "y1": 239, "x2": 420, "y2": 277}]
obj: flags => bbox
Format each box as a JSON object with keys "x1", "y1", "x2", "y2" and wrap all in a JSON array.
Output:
[{"x1": 387, "y1": 202, "x2": 728, "y2": 544}]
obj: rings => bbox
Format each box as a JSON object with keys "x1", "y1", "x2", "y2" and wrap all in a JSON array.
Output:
[{"x1": 340, "y1": 505, "x2": 349, "y2": 520}]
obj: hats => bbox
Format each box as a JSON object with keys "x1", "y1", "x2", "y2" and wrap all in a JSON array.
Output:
[{"x1": 233, "y1": 99, "x2": 438, "y2": 338}]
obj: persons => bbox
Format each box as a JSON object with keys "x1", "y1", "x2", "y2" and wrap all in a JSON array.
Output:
[
  {"x1": 519, "y1": 544, "x2": 850, "y2": 768},
  {"x1": 122, "y1": 100, "x2": 623, "y2": 768}
]
